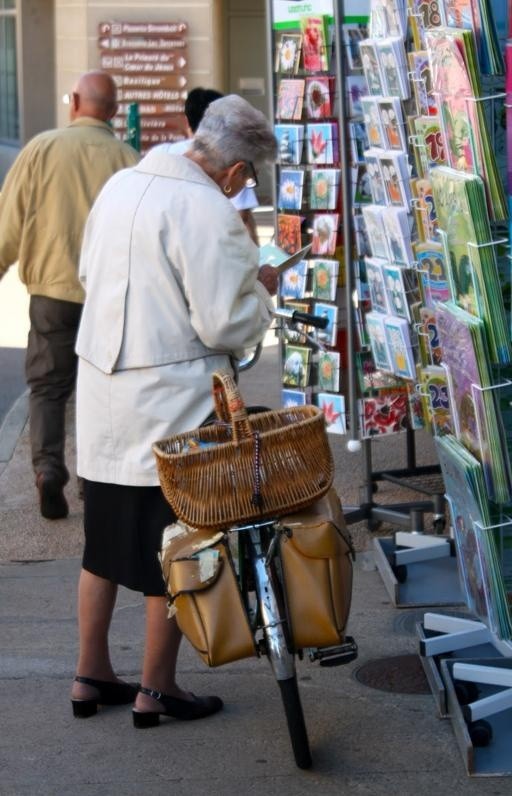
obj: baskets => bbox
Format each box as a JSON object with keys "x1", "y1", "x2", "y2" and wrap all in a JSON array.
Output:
[{"x1": 150, "y1": 368, "x2": 337, "y2": 532}]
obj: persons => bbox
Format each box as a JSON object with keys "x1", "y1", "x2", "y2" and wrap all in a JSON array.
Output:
[
  {"x1": 186, "y1": 85, "x2": 259, "y2": 248},
  {"x1": 0, "y1": 70, "x2": 140, "y2": 519},
  {"x1": 70, "y1": 90, "x2": 281, "y2": 727}
]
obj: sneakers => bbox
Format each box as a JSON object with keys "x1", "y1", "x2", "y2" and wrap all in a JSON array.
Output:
[{"x1": 37, "y1": 471, "x2": 69, "y2": 521}]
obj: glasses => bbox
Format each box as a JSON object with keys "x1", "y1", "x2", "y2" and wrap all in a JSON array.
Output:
[{"x1": 245, "y1": 161, "x2": 260, "y2": 189}]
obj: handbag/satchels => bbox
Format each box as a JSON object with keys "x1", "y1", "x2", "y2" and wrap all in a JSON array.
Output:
[
  {"x1": 272, "y1": 484, "x2": 358, "y2": 649},
  {"x1": 159, "y1": 516, "x2": 260, "y2": 667}
]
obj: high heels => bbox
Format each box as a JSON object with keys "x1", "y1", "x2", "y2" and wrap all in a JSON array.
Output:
[
  {"x1": 132, "y1": 686, "x2": 225, "y2": 729},
  {"x1": 69, "y1": 673, "x2": 143, "y2": 718}
]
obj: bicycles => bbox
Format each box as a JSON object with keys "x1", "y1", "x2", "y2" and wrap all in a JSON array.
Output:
[{"x1": 221, "y1": 304, "x2": 360, "y2": 769}]
